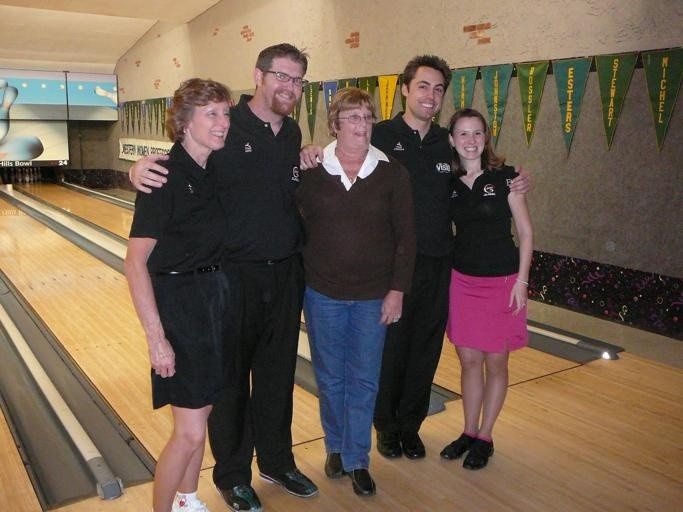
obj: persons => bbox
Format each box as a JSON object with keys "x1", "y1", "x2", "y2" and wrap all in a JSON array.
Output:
[
  {"x1": 123, "y1": 77, "x2": 236, "y2": 512},
  {"x1": 128, "y1": 40, "x2": 321, "y2": 511},
  {"x1": 299, "y1": 54, "x2": 534, "y2": 461},
  {"x1": 294, "y1": 86, "x2": 419, "y2": 497},
  {"x1": 439, "y1": 107, "x2": 535, "y2": 472}
]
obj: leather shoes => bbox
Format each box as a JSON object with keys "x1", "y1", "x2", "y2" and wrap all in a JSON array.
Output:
[
  {"x1": 400, "y1": 432, "x2": 425, "y2": 459},
  {"x1": 346, "y1": 469, "x2": 376, "y2": 496},
  {"x1": 324, "y1": 451, "x2": 345, "y2": 478},
  {"x1": 376, "y1": 430, "x2": 401, "y2": 458}
]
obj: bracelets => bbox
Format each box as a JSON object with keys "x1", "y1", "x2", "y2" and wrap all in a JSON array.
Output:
[{"x1": 515, "y1": 278, "x2": 528, "y2": 285}]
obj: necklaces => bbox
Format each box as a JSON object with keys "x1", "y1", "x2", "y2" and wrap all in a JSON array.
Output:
[{"x1": 349, "y1": 177, "x2": 353, "y2": 182}]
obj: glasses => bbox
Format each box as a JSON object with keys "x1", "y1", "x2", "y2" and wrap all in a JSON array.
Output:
[
  {"x1": 261, "y1": 70, "x2": 303, "y2": 86},
  {"x1": 336, "y1": 112, "x2": 376, "y2": 125}
]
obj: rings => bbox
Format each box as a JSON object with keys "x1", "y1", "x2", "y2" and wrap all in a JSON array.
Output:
[
  {"x1": 304, "y1": 159, "x2": 310, "y2": 162},
  {"x1": 394, "y1": 318, "x2": 397, "y2": 322}
]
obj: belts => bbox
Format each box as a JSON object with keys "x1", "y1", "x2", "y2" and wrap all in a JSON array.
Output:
[
  {"x1": 262, "y1": 256, "x2": 288, "y2": 266},
  {"x1": 166, "y1": 264, "x2": 219, "y2": 276}
]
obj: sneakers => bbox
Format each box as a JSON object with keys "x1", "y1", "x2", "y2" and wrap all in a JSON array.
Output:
[
  {"x1": 439, "y1": 433, "x2": 476, "y2": 459},
  {"x1": 462, "y1": 437, "x2": 494, "y2": 469},
  {"x1": 258, "y1": 464, "x2": 319, "y2": 498},
  {"x1": 214, "y1": 476, "x2": 262, "y2": 512},
  {"x1": 171, "y1": 488, "x2": 210, "y2": 512}
]
